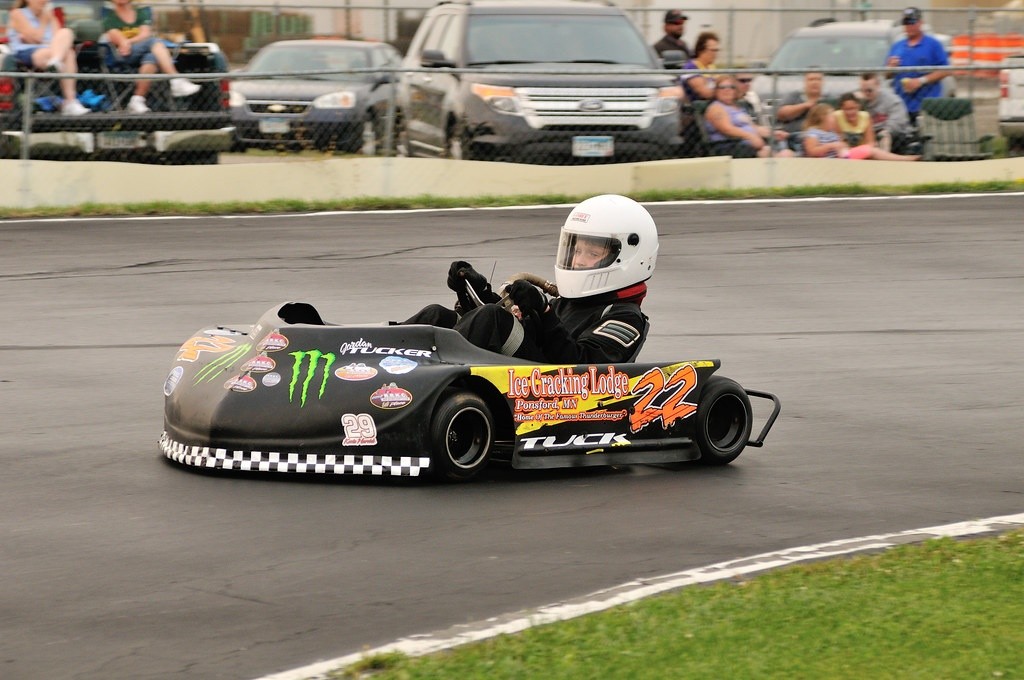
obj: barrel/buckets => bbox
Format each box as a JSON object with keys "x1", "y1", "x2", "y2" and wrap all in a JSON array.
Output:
[{"x1": 946, "y1": 31, "x2": 1024, "y2": 76}]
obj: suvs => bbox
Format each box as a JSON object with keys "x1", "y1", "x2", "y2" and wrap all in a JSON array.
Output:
[
  {"x1": 742, "y1": 18, "x2": 951, "y2": 134},
  {"x1": 394, "y1": 0, "x2": 686, "y2": 167}
]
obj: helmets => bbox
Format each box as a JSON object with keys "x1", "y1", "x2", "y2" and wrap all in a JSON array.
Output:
[{"x1": 555, "y1": 194, "x2": 658, "y2": 297}]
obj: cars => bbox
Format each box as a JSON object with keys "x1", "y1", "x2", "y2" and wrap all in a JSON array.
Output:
[
  {"x1": 0, "y1": 40, "x2": 239, "y2": 168},
  {"x1": 226, "y1": 38, "x2": 403, "y2": 157}
]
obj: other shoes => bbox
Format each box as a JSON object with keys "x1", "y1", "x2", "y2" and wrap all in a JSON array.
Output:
[
  {"x1": 60, "y1": 100, "x2": 92, "y2": 116},
  {"x1": 169, "y1": 78, "x2": 203, "y2": 98},
  {"x1": 128, "y1": 96, "x2": 151, "y2": 113},
  {"x1": 47, "y1": 60, "x2": 61, "y2": 72}
]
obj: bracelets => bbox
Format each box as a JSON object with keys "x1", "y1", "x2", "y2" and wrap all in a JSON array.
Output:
[{"x1": 919, "y1": 78, "x2": 927, "y2": 84}]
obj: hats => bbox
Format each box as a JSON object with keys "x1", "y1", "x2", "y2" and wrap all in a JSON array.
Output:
[
  {"x1": 903, "y1": 7, "x2": 922, "y2": 20},
  {"x1": 666, "y1": 10, "x2": 687, "y2": 22}
]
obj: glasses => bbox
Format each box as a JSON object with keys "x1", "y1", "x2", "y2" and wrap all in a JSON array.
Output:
[
  {"x1": 738, "y1": 78, "x2": 753, "y2": 82},
  {"x1": 902, "y1": 18, "x2": 916, "y2": 25},
  {"x1": 719, "y1": 84, "x2": 736, "y2": 89},
  {"x1": 673, "y1": 20, "x2": 684, "y2": 25}
]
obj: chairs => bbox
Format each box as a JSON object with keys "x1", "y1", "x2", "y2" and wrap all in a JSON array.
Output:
[
  {"x1": 0, "y1": 40, "x2": 121, "y2": 117},
  {"x1": 913, "y1": 96, "x2": 996, "y2": 161},
  {"x1": 98, "y1": 39, "x2": 181, "y2": 114},
  {"x1": 693, "y1": 98, "x2": 735, "y2": 156}
]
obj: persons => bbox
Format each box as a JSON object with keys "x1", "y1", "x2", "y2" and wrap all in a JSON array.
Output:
[
  {"x1": 798, "y1": 102, "x2": 922, "y2": 161},
  {"x1": 882, "y1": 7, "x2": 952, "y2": 124},
  {"x1": 678, "y1": 33, "x2": 720, "y2": 102},
  {"x1": 397, "y1": 194, "x2": 659, "y2": 365},
  {"x1": 777, "y1": 64, "x2": 829, "y2": 151},
  {"x1": 852, "y1": 74, "x2": 919, "y2": 152},
  {"x1": 832, "y1": 93, "x2": 875, "y2": 149},
  {"x1": 703, "y1": 75, "x2": 796, "y2": 158},
  {"x1": 104, "y1": 0, "x2": 202, "y2": 112},
  {"x1": 732, "y1": 72, "x2": 795, "y2": 157},
  {"x1": 7, "y1": 0, "x2": 92, "y2": 116},
  {"x1": 652, "y1": 9, "x2": 688, "y2": 57}
]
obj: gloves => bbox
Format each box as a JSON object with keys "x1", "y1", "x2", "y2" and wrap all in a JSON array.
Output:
[
  {"x1": 505, "y1": 280, "x2": 548, "y2": 318},
  {"x1": 448, "y1": 261, "x2": 487, "y2": 293}
]
